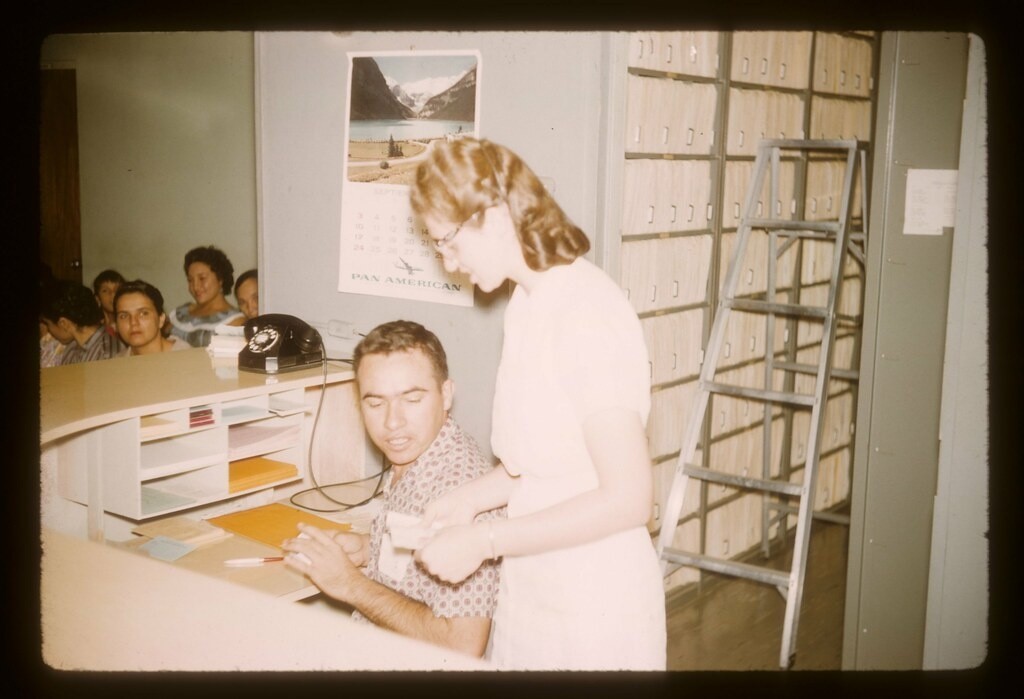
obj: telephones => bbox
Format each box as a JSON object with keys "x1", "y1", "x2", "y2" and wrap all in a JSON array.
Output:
[{"x1": 235, "y1": 306, "x2": 325, "y2": 376}]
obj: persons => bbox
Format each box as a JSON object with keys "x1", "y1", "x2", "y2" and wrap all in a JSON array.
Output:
[
  {"x1": 407, "y1": 131, "x2": 669, "y2": 672},
  {"x1": 40, "y1": 245, "x2": 261, "y2": 371},
  {"x1": 282, "y1": 316, "x2": 503, "y2": 662}
]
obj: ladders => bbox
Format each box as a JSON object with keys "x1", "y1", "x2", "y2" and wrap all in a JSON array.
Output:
[{"x1": 653, "y1": 133, "x2": 876, "y2": 671}]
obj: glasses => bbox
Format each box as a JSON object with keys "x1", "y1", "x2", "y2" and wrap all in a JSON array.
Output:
[{"x1": 431, "y1": 201, "x2": 504, "y2": 260}]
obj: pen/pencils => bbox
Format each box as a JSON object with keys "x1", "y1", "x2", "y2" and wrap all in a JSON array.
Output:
[{"x1": 221, "y1": 553, "x2": 287, "y2": 568}]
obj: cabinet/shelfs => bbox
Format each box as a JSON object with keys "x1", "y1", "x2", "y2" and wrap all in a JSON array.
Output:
[{"x1": 622, "y1": 28, "x2": 881, "y2": 613}]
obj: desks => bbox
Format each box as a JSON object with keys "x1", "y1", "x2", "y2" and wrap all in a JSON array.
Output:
[{"x1": 117, "y1": 479, "x2": 386, "y2": 602}]
